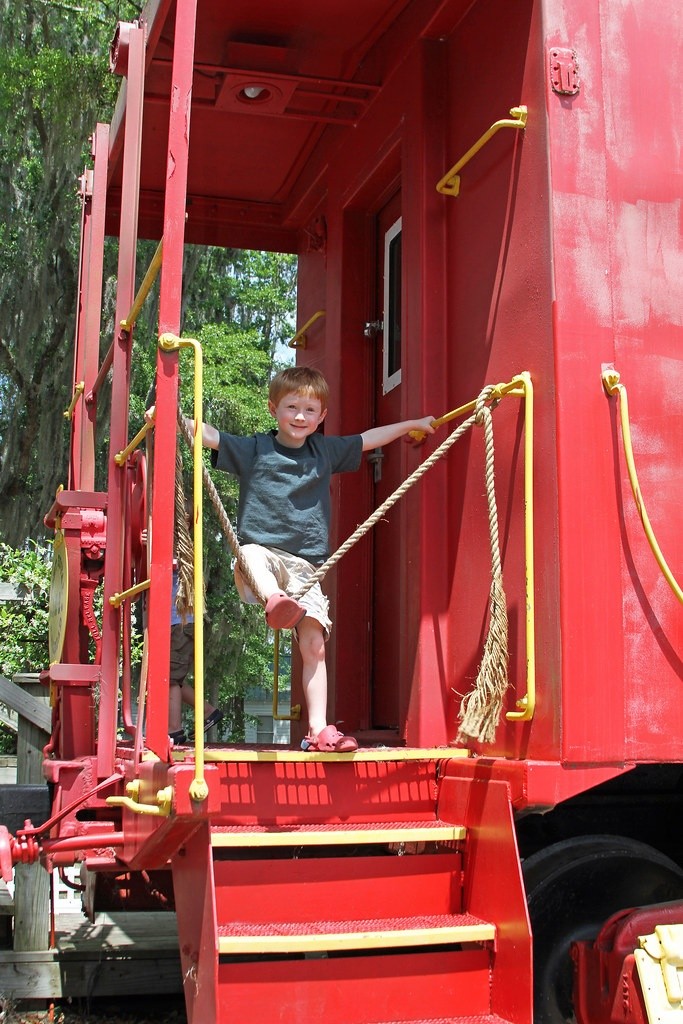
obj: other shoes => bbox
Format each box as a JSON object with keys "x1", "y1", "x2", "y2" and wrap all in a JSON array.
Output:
[
  {"x1": 188, "y1": 710, "x2": 223, "y2": 739},
  {"x1": 170, "y1": 732, "x2": 186, "y2": 745}
]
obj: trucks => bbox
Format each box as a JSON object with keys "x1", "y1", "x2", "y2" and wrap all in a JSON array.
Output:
[{"x1": 1, "y1": 0, "x2": 683, "y2": 1023}]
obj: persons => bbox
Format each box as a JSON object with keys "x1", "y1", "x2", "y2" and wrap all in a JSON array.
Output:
[
  {"x1": 143, "y1": 367, "x2": 439, "y2": 751},
  {"x1": 141, "y1": 494, "x2": 223, "y2": 745}
]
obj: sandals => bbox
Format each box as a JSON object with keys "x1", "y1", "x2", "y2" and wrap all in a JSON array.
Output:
[
  {"x1": 302, "y1": 723, "x2": 359, "y2": 752},
  {"x1": 265, "y1": 594, "x2": 307, "y2": 627}
]
